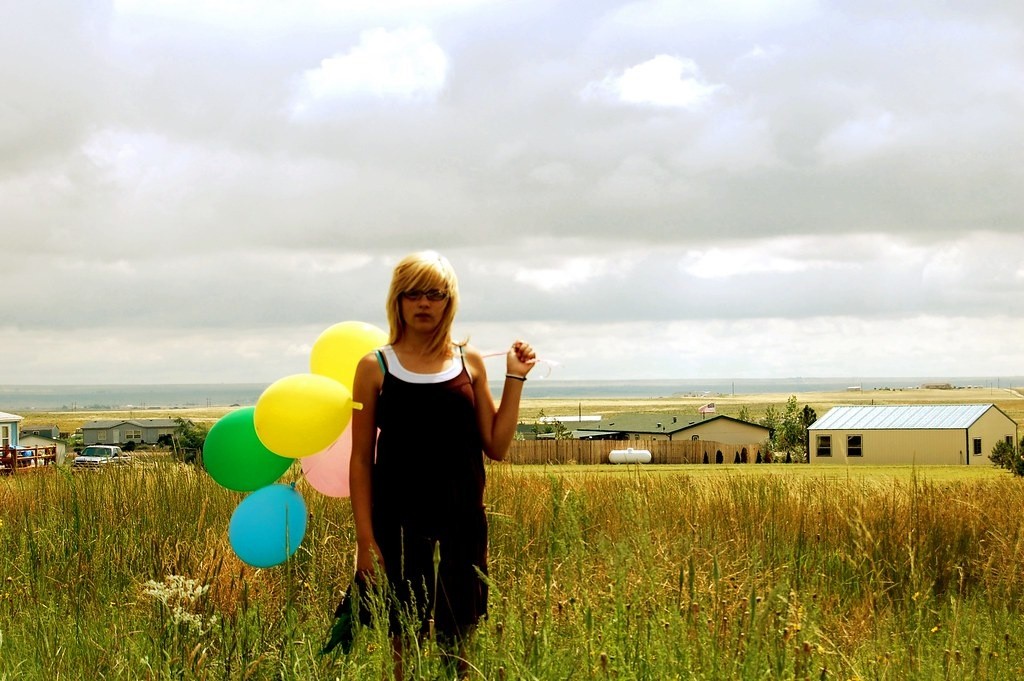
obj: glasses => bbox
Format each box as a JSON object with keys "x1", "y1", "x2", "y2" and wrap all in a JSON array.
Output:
[{"x1": 402, "y1": 289, "x2": 447, "y2": 301}]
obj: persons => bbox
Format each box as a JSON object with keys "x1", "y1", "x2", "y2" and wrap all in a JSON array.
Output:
[{"x1": 319, "y1": 250, "x2": 536, "y2": 681}]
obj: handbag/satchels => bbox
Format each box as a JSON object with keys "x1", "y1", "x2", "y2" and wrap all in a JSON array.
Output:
[{"x1": 325, "y1": 567, "x2": 391, "y2": 655}]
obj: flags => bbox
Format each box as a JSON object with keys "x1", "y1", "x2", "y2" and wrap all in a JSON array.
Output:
[{"x1": 699, "y1": 403, "x2": 715, "y2": 413}]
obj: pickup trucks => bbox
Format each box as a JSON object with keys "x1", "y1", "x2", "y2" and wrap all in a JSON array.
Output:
[{"x1": 72, "y1": 446, "x2": 134, "y2": 467}]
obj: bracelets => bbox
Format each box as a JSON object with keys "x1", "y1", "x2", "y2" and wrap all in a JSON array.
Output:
[{"x1": 506, "y1": 374, "x2": 526, "y2": 381}]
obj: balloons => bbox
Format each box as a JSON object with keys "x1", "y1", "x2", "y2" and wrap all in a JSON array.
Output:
[
  {"x1": 254, "y1": 374, "x2": 363, "y2": 458},
  {"x1": 301, "y1": 419, "x2": 380, "y2": 497},
  {"x1": 227, "y1": 481, "x2": 307, "y2": 568},
  {"x1": 203, "y1": 407, "x2": 294, "y2": 491},
  {"x1": 310, "y1": 322, "x2": 389, "y2": 395}
]
